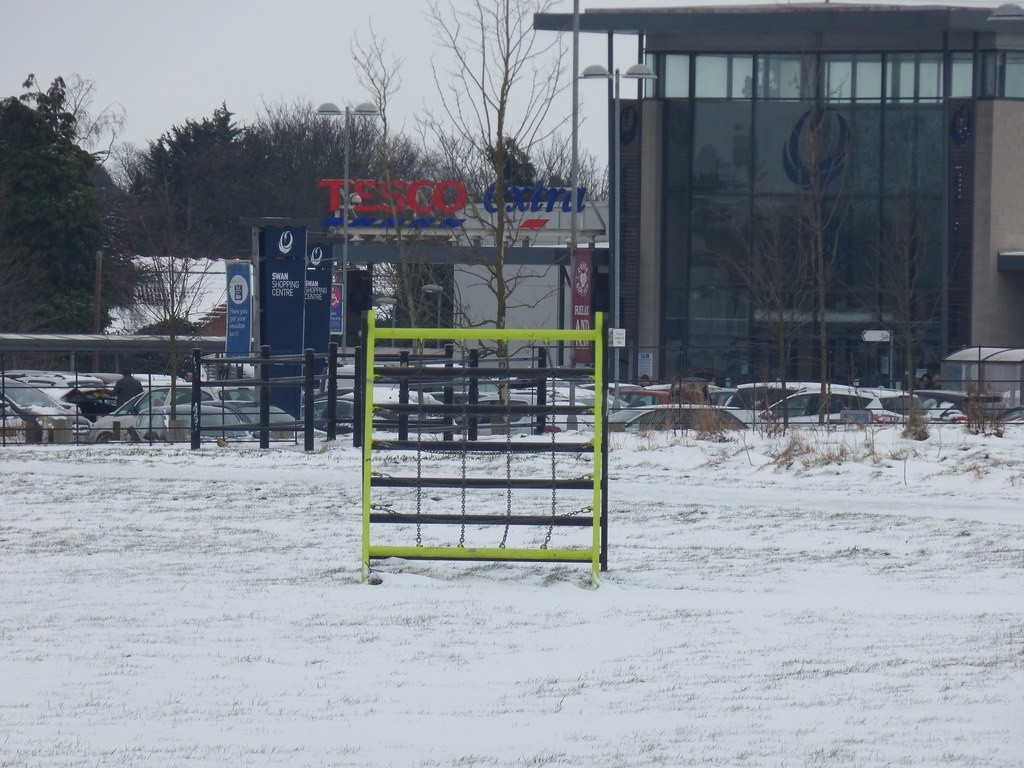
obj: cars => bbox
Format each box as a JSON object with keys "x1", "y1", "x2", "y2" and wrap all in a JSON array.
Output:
[
  {"x1": 572, "y1": 403, "x2": 773, "y2": 433},
  {"x1": 1, "y1": 360, "x2": 732, "y2": 443}
]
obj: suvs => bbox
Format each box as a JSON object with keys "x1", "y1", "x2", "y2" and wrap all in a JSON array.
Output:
[
  {"x1": 724, "y1": 382, "x2": 852, "y2": 409},
  {"x1": 906, "y1": 389, "x2": 1013, "y2": 424},
  {"x1": 745, "y1": 387, "x2": 929, "y2": 428}
]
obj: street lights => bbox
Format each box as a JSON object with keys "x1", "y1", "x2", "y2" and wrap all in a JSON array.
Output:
[
  {"x1": 313, "y1": 102, "x2": 383, "y2": 372},
  {"x1": 578, "y1": 64, "x2": 660, "y2": 415}
]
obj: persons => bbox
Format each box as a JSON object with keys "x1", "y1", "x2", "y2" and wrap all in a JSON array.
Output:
[
  {"x1": 102, "y1": 368, "x2": 144, "y2": 408},
  {"x1": 185, "y1": 372, "x2": 193, "y2": 383},
  {"x1": 640, "y1": 375, "x2": 653, "y2": 388}
]
